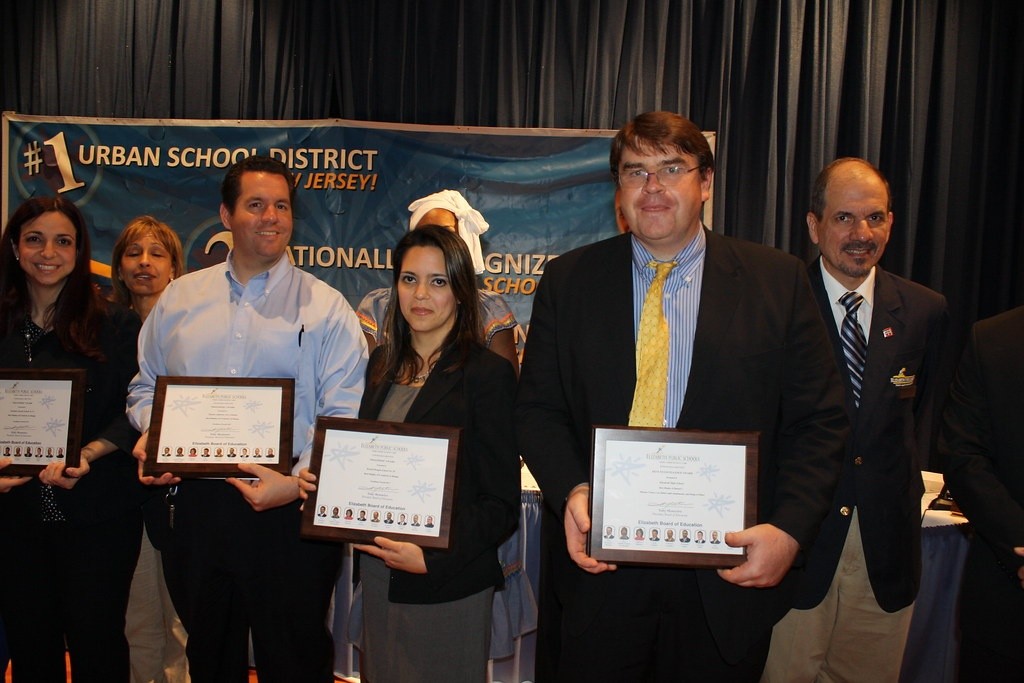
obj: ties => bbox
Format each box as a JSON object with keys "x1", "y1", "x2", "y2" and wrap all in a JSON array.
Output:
[
  {"x1": 837, "y1": 291, "x2": 867, "y2": 410},
  {"x1": 628, "y1": 260, "x2": 679, "y2": 430}
]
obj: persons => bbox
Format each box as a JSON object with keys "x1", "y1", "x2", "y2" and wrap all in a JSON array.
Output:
[
  {"x1": 125, "y1": 153, "x2": 369, "y2": 683},
  {"x1": 162, "y1": 448, "x2": 274, "y2": 458},
  {"x1": 760, "y1": 158, "x2": 949, "y2": 683},
  {"x1": 111, "y1": 217, "x2": 187, "y2": 637},
  {"x1": 317, "y1": 506, "x2": 434, "y2": 527},
  {"x1": 604, "y1": 527, "x2": 721, "y2": 544},
  {"x1": 943, "y1": 304, "x2": 1024, "y2": 683},
  {"x1": 3, "y1": 447, "x2": 64, "y2": 458},
  {"x1": 0, "y1": 196, "x2": 144, "y2": 683},
  {"x1": 346, "y1": 190, "x2": 537, "y2": 658}
]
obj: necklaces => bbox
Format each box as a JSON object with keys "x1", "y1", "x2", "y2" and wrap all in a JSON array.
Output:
[{"x1": 407, "y1": 360, "x2": 436, "y2": 383}]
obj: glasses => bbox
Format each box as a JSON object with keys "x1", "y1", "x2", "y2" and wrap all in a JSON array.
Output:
[{"x1": 617, "y1": 165, "x2": 703, "y2": 189}]
